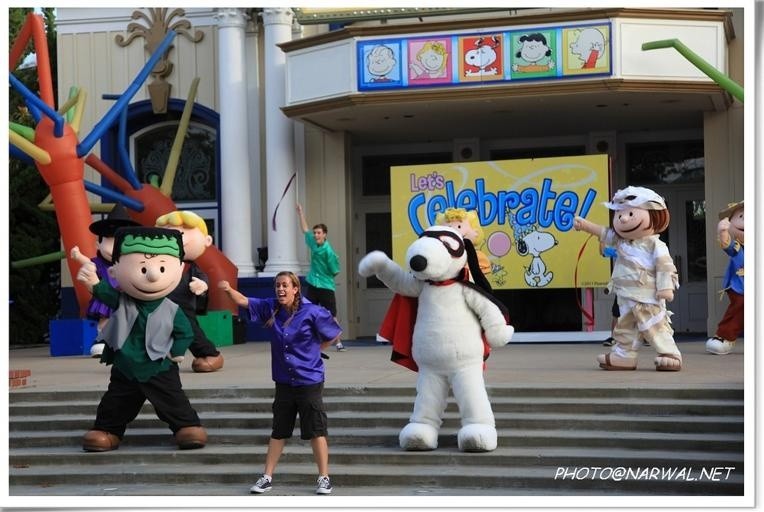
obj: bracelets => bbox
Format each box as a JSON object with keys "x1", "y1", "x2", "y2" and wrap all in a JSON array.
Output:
[{"x1": 224, "y1": 287, "x2": 231, "y2": 291}]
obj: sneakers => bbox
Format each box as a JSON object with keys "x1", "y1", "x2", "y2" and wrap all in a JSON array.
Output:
[
  {"x1": 315, "y1": 474, "x2": 334, "y2": 493},
  {"x1": 249, "y1": 476, "x2": 273, "y2": 495},
  {"x1": 602, "y1": 336, "x2": 616, "y2": 348},
  {"x1": 336, "y1": 343, "x2": 347, "y2": 353}
]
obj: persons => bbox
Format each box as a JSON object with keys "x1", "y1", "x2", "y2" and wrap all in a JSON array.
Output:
[
  {"x1": 705, "y1": 199, "x2": 744, "y2": 356},
  {"x1": 433, "y1": 206, "x2": 491, "y2": 372},
  {"x1": 573, "y1": 185, "x2": 682, "y2": 372},
  {"x1": 294, "y1": 203, "x2": 344, "y2": 352},
  {"x1": 358, "y1": 224, "x2": 515, "y2": 452},
  {"x1": 75, "y1": 225, "x2": 207, "y2": 452},
  {"x1": 69, "y1": 200, "x2": 144, "y2": 357},
  {"x1": 599, "y1": 241, "x2": 651, "y2": 347},
  {"x1": 155, "y1": 209, "x2": 224, "y2": 373},
  {"x1": 215, "y1": 271, "x2": 343, "y2": 495}
]
